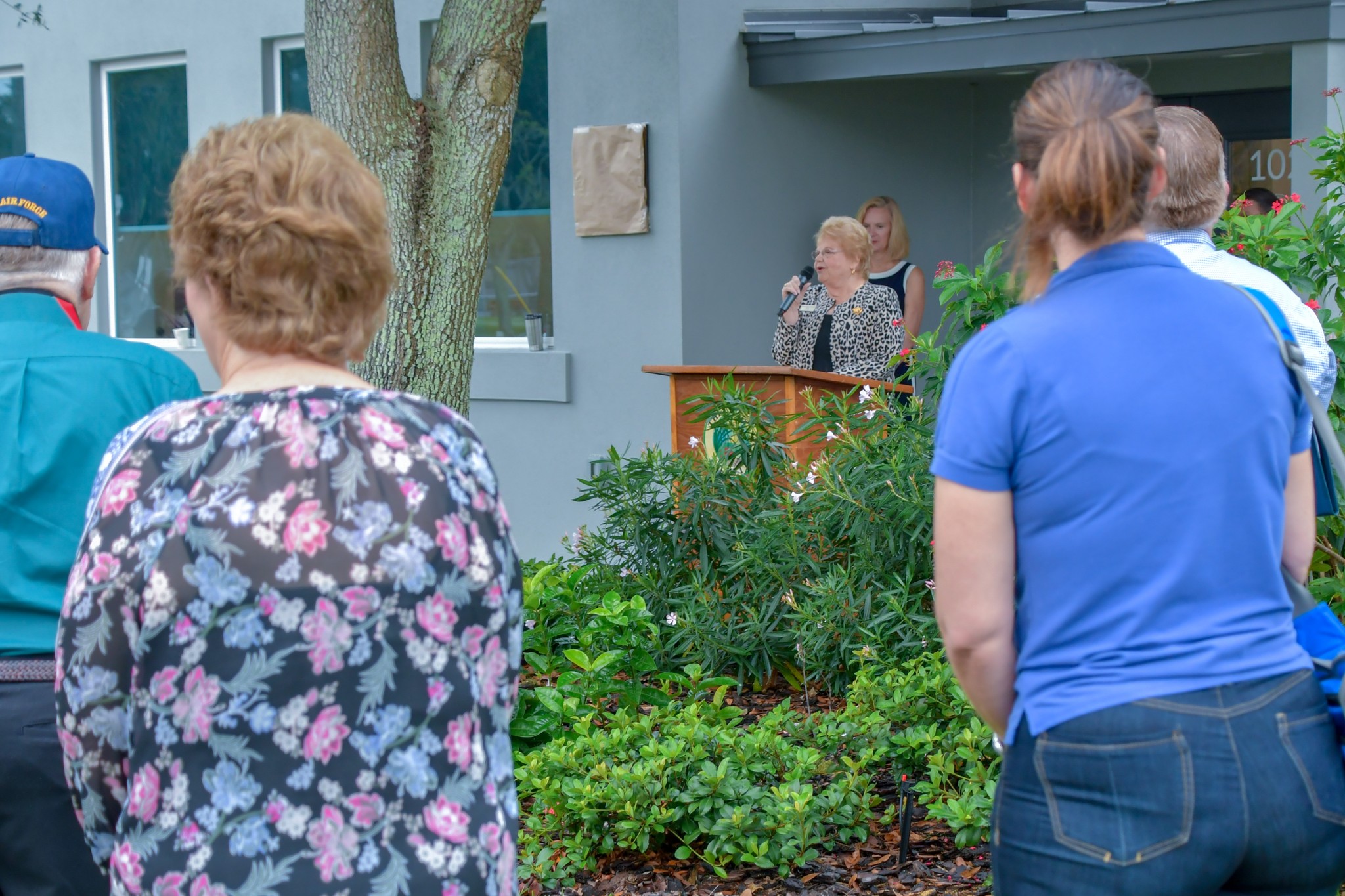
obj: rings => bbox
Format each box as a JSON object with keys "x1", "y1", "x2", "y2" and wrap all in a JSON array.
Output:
[{"x1": 784, "y1": 282, "x2": 788, "y2": 286}]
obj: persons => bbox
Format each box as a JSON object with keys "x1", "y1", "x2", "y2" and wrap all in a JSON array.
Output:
[
  {"x1": 928, "y1": 54, "x2": 1342, "y2": 894},
  {"x1": 856, "y1": 195, "x2": 930, "y2": 435},
  {"x1": 51, "y1": 110, "x2": 528, "y2": 896},
  {"x1": 0, "y1": 153, "x2": 205, "y2": 894},
  {"x1": 1146, "y1": 103, "x2": 1337, "y2": 451},
  {"x1": 774, "y1": 219, "x2": 905, "y2": 396},
  {"x1": 1224, "y1": 182, "x2": 1342, "y2": 328}
]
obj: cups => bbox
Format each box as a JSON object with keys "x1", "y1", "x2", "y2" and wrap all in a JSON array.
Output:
[
  {"x1": 525, "y1": 313, "x2": 543, "y2": 352},
  {"x1": 172, "y1": 328, "x2": 190, "y2": 350}
]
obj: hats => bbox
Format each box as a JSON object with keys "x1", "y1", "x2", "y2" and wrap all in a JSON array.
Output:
[{"x1": 1, "y1": 152, "x2": 109, "y2": 254}]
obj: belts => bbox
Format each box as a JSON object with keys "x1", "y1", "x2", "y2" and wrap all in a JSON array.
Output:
[{"x1": 0, "y1": 659, "x2": 56, "y2": 681}]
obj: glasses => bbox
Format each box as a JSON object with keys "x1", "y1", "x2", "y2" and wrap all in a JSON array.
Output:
[{"x1": 811, "y1": 248, "x2": 844, "y2": 260}]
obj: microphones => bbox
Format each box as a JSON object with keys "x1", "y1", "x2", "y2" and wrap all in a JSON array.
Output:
[{"x1": 776, "y1": 265, "x2": 815, "y2": 317}]
